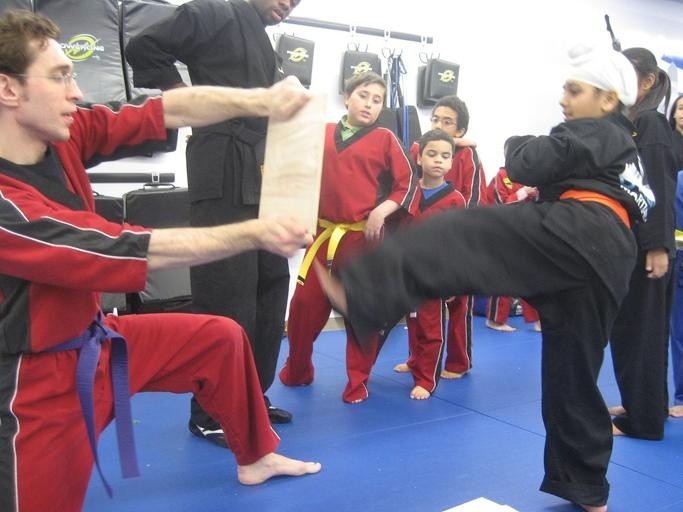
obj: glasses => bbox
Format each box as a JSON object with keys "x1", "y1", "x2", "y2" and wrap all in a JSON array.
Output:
[
  {"x1": 431, "y1": 117, "x2": 459, "y2": 127},
  {"x1": 3, "y1": 71, "x2": 79, "y2": 89}
]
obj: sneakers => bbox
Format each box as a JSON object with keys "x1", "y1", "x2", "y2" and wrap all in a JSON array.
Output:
[
  {"x1": 188, "y1": 415, "x2": 228, "y2": 449},
  {"x1": 266, "y1": 397, "x2": 295, "y2": 425}
]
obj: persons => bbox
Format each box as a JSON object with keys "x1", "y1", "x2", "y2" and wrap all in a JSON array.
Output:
[
  {"x1": 667, "y1": 169, "x2": 682, "y2": 421},
  {"x1": 667, "y1": 91, "x2": 682, "y2": 134},
  {"x1": 317, "y1": 46, "x2": 657, "y2": 512},
  {"x1": 485, "y1": 135, "x2": 542, "y2": 335},
  {"x1": 370, "y1": 130, "x2": 469, "y2": 402},
  {"x1": 605, "y1": 47, "x2": 682, "y2": 443},
  {"x1": 391, "y1": 92, "x2": 488, "y2": 383},
  {"x1": 119, "y1": 0, "x2": 302, "y2": 454},
  {"x1": 276, "y1": 70, "x2": 417, "y2": 409},
  {"x1": 0, "y1": 10, "x2": 326, "y2": 511}
]
